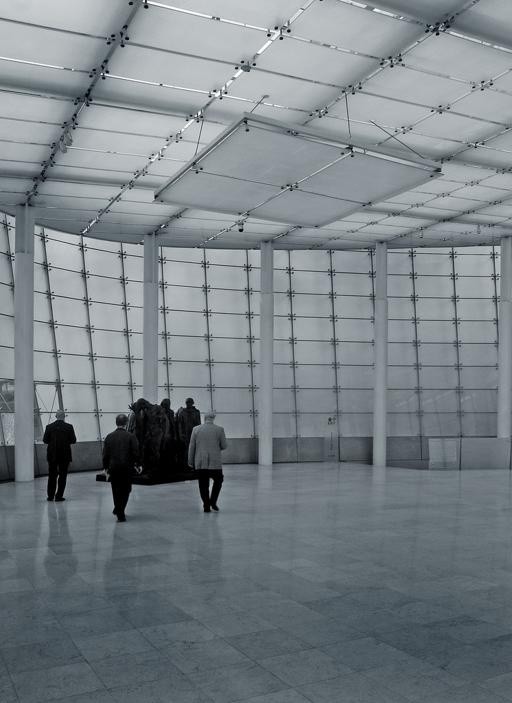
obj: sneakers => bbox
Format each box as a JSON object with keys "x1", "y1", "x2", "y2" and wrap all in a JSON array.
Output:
[{"x1": 46, "y1": 495, "x2": 220, "y2": 521}]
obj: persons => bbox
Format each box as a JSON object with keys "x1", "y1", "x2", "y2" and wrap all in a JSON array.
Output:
[
  {"x1": 131, "y1": 396, "x2": 201, "y2": 474},
  {"x1": 101, "y1": 414, "x2": 145, "y2": 522},
  {"x1": 42, "y1": 409, "x2": 77, "y2": 502},
  {"x1": 187, "y1": 410, "x2": 228, "y2": 512}
]
